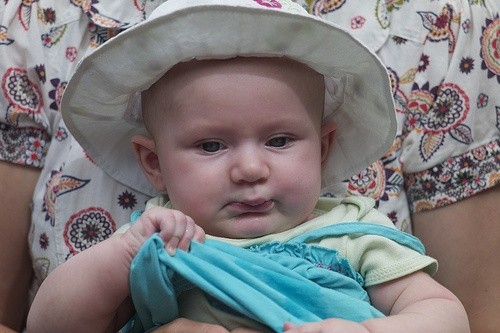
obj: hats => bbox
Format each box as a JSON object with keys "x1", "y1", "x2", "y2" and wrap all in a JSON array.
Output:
[{"x1": 61, "y1": 0, "x2": 397, "y2": 198}]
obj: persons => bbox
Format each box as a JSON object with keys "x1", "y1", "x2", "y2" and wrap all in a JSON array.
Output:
[
  {"x1": 27, "y1": 0, "x2": 471, "y2": 333},
  {"x1": 0, "y1": 0, "x2": 500, "y2": 333}
]
